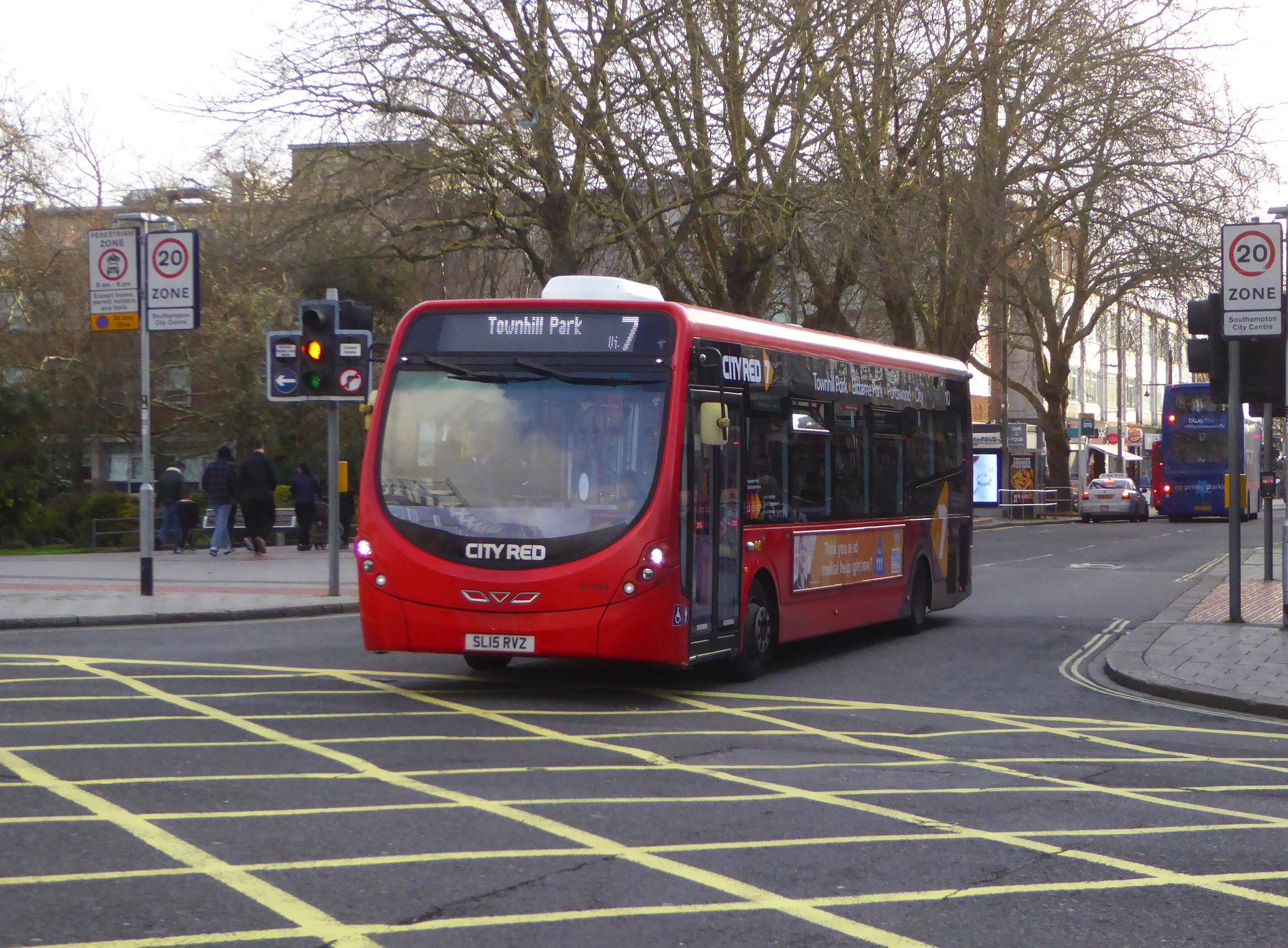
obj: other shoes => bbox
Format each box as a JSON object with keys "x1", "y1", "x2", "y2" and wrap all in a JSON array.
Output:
[
  {"x1": 298, "y1": 544, "x2": 312, "y2": 551},
  {"x1": 173, "y1": 547, "x2": 184, "y2": 553},
  {"x1": 224, "y1": 548, "x2": 235, "y2": 554},
  {"x1": 256, "y1": 538, "x2": 266, "y2": 554},
  {"x1": 244, "y1": 536, "x2": 255, "y2": 550},
  {"x1": 154, "y1": 537, "x2": 162, "y2": 551},
  {"x1": 209, "y1": 549, "x2": 221, "y2": 557}
]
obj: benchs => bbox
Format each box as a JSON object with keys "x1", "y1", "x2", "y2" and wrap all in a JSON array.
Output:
[{"x1": 202, "y1": 508, "x2": 297, "y2": 546}]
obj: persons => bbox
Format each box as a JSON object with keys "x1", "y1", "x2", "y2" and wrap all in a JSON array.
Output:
[
  {"x1": 155, "y1": 462, "x2": 199, "y2": 553},
  {"x1": 338, "y1": 484, "x2": 355, "y2": 551},
  {"x1": 286, "y1": 462, "x2": 321, "y2": 552},
  {"x1": 1140, "y1": 471, "x2": 1150, "y2": 493},
  {"x1": 202, "y1": 440, "x2": 278, "y2": 557},
  {"x1": 452, "y1": 430, "x2": 492, "y2": 486}
]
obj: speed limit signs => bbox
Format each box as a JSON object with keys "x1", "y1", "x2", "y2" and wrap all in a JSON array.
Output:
[
  {"x1": 1230, "y1": 230, "x2": 1277, "y2": 276},
  {"x1": 153, "y1": 238, "x2": 188, "y2": 279}
]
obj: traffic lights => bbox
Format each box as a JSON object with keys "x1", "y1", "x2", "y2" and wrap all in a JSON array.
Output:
[
  {"x1": 300, "y1": 301, "x2": 339, "y2": 400},
  {"x1": 1185, "y1": 291, "x2": 1231, "y2": 405}
]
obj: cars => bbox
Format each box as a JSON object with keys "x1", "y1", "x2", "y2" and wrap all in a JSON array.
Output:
[{"x1": 1080, "y1": 478, "x2": 1153, "y2": 522}]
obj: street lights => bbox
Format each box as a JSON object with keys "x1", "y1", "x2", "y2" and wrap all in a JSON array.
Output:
[{"x1": 111, "y1": 210, "x2": 175, "y2": 596}]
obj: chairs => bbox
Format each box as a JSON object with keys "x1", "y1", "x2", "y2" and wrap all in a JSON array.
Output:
[{"x1": 744, "y1": 460, "x2": 944, "y2": 524}]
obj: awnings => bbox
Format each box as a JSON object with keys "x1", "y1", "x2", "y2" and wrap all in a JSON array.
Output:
[{"x1": 1091, "y1": 444, "x2": 1143, "y2": 460}]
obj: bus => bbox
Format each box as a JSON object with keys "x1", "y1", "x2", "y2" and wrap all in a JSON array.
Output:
[
  {"x1": 1161, "y1": 382, "x2": 1262, "y2": 523},
  {"x1": 1152, "y1": 440, "x2": 1168, "y2": 514},
  {"x1": 355, "y1": 273, "x2": 978, "y2": 683}
]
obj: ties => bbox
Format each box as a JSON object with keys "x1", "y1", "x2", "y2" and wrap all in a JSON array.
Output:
[{"x1": 474, "y1": 460, "x2": 480, "y2": 469}]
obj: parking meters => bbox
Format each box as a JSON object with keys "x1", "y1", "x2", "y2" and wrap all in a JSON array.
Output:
[{"x1": 1259, "y1": 471, "x2": 1277, "y2": 581}]
